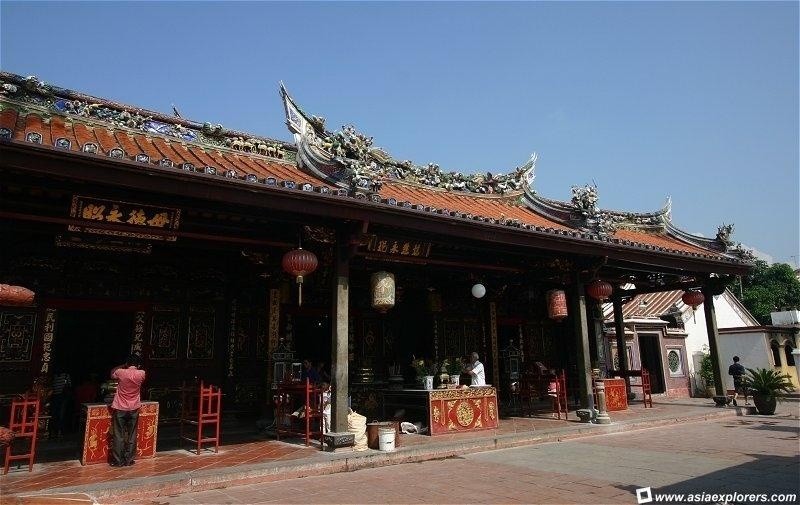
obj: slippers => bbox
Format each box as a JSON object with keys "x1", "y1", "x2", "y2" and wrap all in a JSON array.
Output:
[
  {"x1": 745, "y1": 403, "x2": 751, "y2": 405},
  {"x1": 733, "y1": 398, "x2": 737, "y2": 406}
]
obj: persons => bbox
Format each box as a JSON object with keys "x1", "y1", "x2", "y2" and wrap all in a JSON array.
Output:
[
  {"x1": 463, "y1": 352, "x2": 488, "y2": 386},
  {"x1": 109, "y1": 354, "x2": 146, "y2": 466},
  {"x1": 728, "y1": 355, "x2": 751, "y2": 407},
  {"x1": 45, "y1": 364, "x2": 72, "y2": 423},
  {"x1": 75, "y1": 374, "x2": 98, "y2": 432},
  {"x1": 300, "y1": 358, "x2": 321, "y2": 385}
]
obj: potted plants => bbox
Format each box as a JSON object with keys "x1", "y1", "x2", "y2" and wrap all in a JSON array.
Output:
[
  {"x1": 416, "y1": 360, "x2": 461, "y2": 390},
  {"x1": 695, "y1": 354, "x2": 717, "y2": 399},
  {"x1": 735, "y1": 367, "x2": 797, "y2": 415}
]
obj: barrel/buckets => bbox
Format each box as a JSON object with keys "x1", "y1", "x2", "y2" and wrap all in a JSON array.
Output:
[{"x1": 379, "y1": 428, "x2": 397, "y2": 452}]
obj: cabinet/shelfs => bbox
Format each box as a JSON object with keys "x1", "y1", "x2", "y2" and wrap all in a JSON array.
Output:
[
  {"x1": 3, "y1": 390, "x2": 40, "y2": 475},
  {"x1": 518, "y1": 367, "x2": 569, "y2": 420},
  {"x1": 275, "y1": 376, "x2": 324, "y2": 447},
  {"x1": 181, "y1": 376, "x2": 221, "y2": 455},
  {"x1": 611, "y1": 366, "x2": 652, "y2": 408}
]
